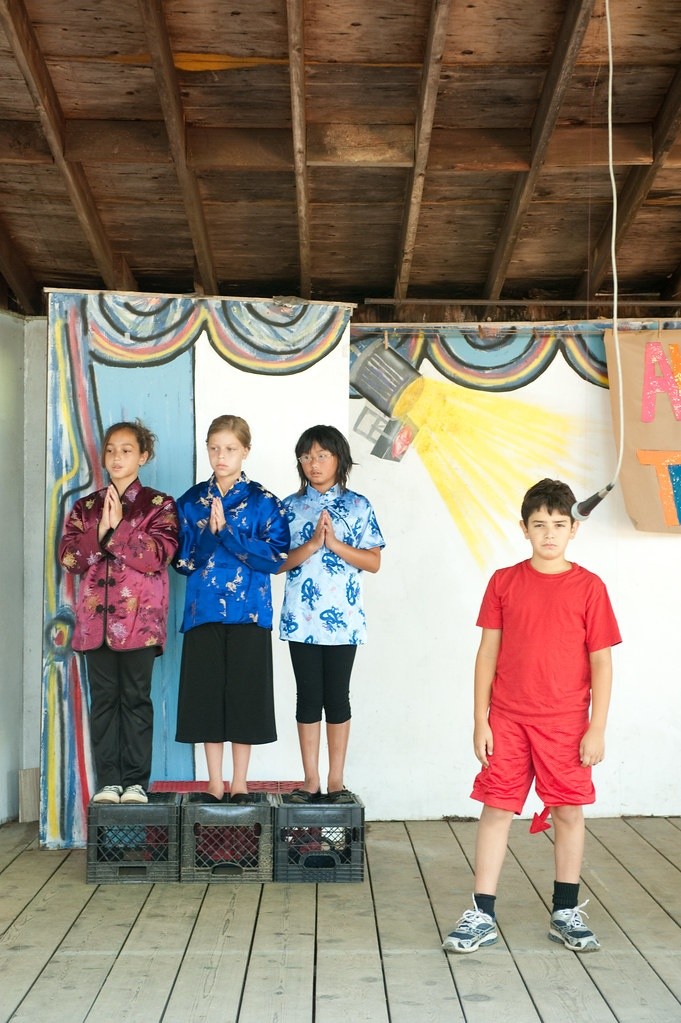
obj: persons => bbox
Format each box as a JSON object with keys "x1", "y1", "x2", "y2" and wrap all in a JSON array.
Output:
[
  {"x1": 442, "y1": 476, "x2": 622, "y2": 954},
  {"x1": 175, "y1": 414, "x2": 291, "y2": 800},
  {"x1": 59, "y1": 422, "x2": 178, "y2": 802},
  {"x1": 276, "y1": 425, "x2": 385, "y2": 804}
]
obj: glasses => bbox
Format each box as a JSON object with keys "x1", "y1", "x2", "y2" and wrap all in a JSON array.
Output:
[{"x1": 298, "y1": 453, "x2": 334, "y2": 465}]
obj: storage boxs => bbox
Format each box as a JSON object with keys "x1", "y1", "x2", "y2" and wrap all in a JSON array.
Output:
[
  {"x1": 234, "y1": 781, "x2": 321, "y2": 861},
  {"x1": 84, "y1": 791, "x2": 180, "y2": 883},
  {"x1": 180, "y1": 790, "x2": 274, "y2": 884},
  {"x1": 146, "y1": 780, "x2": 232, "y2": 862},
  {"x1": 273, "y1": 793, "x2": 366, "y2": 884}
]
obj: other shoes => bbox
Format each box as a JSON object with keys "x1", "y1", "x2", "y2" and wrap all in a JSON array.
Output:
[
  {"x1": 289, "y1": 788, "x2": 321, "y2": 804},
  {"x1": 202, "y1": 793, "x2": 222, "y2": 803},
  {"x1": 328, "y1": 787, "x2": 353, "y2": 804},
  {"x1": 230, "y1": 791, "x2": 252, "y2": 803}
]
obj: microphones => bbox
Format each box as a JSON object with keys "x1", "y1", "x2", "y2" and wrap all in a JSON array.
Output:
[{"x1": 571, "y1": 484, "x2": 615, "y2": 520}]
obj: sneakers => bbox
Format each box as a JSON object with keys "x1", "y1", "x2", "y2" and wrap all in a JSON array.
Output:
[
  {"x1": 121, "y1": 784, "x2": 149, "y2": 804},
  {"x1": 548, "y1": 899, "x2": 599, "y2": 951},
  {"x1": 441, "y1": 892, "x2": 498, "y2": 953},
  {"x1": 93, "y1": 783, "x2": 123, "y2": 804}
]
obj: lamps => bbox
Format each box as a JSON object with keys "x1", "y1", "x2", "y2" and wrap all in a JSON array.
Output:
[{"x1": 350, "y1": 330, "x2": 425, "y2": 418}]
